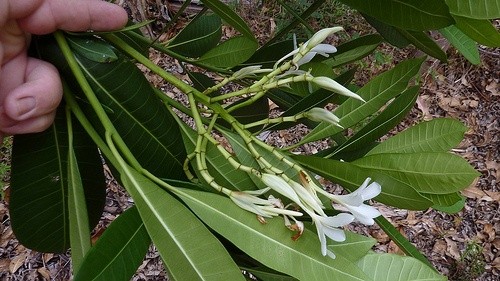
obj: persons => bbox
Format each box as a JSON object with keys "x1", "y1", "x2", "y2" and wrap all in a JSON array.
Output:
[{"x1": 0, "y1": 0, "x2": 127, "y2": 148}]
